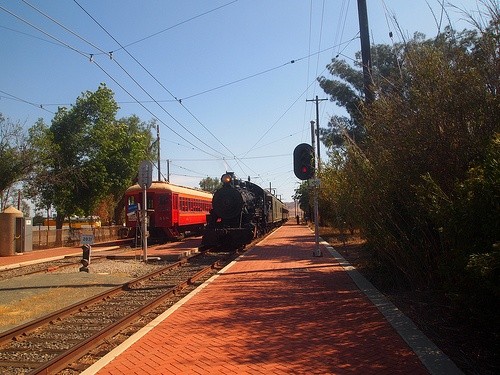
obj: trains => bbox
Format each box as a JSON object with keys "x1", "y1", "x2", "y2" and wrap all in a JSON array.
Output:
[
  {"x1": 203, "y1": 170, "x2": 290, "y2": 245},
  {"x1": 123, "y1": 181, "x2": 214, "y2": 239}
]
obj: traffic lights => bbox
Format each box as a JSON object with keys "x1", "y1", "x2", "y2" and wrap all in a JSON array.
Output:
[
  {"x1": 81, "y1": 243, "x2": 90, "y2": 267},
  {"x1": 295, "y1": 143, "x2": 314, "y2": 180}
]
